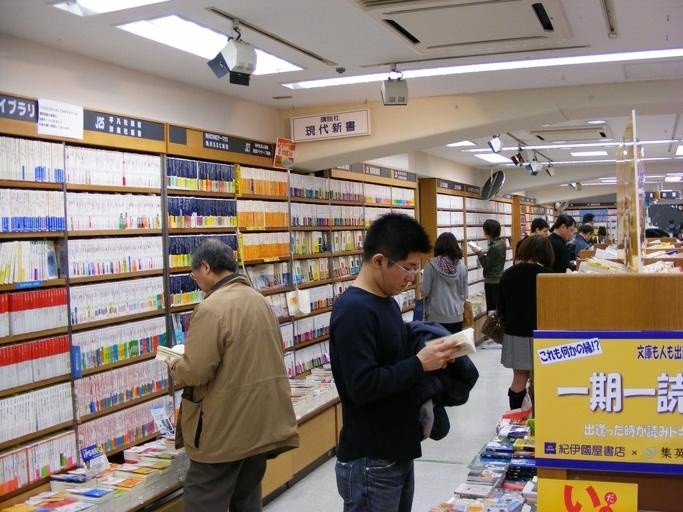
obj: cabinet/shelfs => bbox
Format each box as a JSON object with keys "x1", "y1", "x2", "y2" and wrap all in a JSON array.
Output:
[
  {"x1": 537, "y1": 236, "x2": 683, "y2": 512},
  {"x1": 422, "y1": 178, "x2": 513, "y2": 286},
  {"x1": 0, "y1": 91, "x2": 421, "y2": 512},
  {"x1": 563, "y1": 205, "x2": 617, "y2": 241},
  {"x1": 512, "y1": 196, "x2": 554, "y2": 258}
]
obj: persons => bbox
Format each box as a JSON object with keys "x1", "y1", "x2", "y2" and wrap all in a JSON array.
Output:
[
  {"x1": 667, "y1": 219, "x2": 683, "y2": 242},
  {"x1": 496, "y1": 235, "x2": 555, "y2": 419},
  {"x1": 472, "y1": 219, "x2": 507, "y2": 350},
  {"x1": 420, "y1": 232, "x2": 468, "y2": 334},
  {"x1": 329, "y1": 213, "x2": 479, "y2": 512},
  {"x1": 169, "y1": 239, "x2": 299, "y2": 512},
  {"x1": 515, "y1": 213, "x2": 606, "y2": 273}
]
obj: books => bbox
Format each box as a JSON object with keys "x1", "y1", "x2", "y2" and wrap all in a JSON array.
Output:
[
  {"x1": 432, "y1": 408, "x2": 537, "y2": 512},
  {"x1": 80, "y1": 146, "x2": 239, "y2": 512},
  {"x1": 424, "y1": 328, "x2": 477, "y2": 360},
  {"x1": 520, "y1": 204, "x2": 617, "y2": 244},
  {"x1": 1, "y1": 133, "x2": 81, "y2": 512},
  {"x1": 239, "y1": 136, "x2": 415, "y2": 422},
  {"x1": 566, "y1": 239, "x2": 683, "y2": 274},
  {"x1": 437, "y1": 193, "x2": 513, "y2": 319}
]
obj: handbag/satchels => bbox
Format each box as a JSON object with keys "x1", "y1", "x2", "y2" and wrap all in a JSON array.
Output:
[
  {"x1": 481, "y1": 312, "x2": 504, "y2": 343},
  {"x1": 413, "y1": 297, "x2": 426, "y2": 322},
  {"x1": 464, "y1": 296, "x2": 474, "y2": 326}
]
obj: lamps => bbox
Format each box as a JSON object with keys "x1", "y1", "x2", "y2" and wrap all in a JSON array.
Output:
[
  {"x1": 527, "y1": 156, "x2": 542, "y2": 175},
  {"x1": 488, "y1": 133, "x2": 503, "y2": 153},
  {"x1": 208, "y1": 18, "x2": 257, "y2": 86},
  {"x1": 546, "y1": 164, "x2": 555, "y2": 177},
  {"x1": 381, "y1": 64, "x2": 409, "y2": 106},
  {"x1": 511, "y1": 146, "x2": 527, "y2": 166}
]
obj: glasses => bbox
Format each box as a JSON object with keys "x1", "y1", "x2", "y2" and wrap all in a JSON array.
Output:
[
  {"x1": 386, "y1": 256, "x2": 421, "y2": 276},
  {"x1": 189, "y1": 272, "x2": 196, "y2": 281}
]
